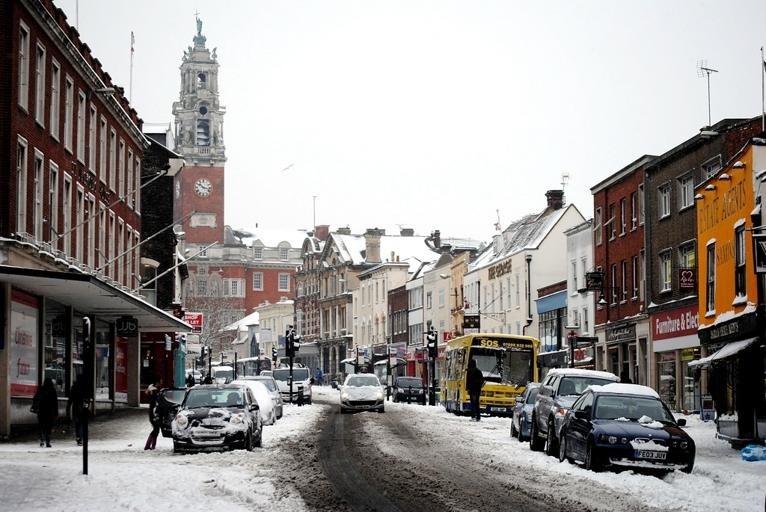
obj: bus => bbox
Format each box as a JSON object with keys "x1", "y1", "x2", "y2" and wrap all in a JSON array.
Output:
[{"x1": 438, "y1": 332, "x2": 542, "y2": 418}]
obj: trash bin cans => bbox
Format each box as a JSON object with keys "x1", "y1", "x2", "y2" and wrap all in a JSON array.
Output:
[{"x1": 699, "y1": 394, "x2": 715, "y2": 420}]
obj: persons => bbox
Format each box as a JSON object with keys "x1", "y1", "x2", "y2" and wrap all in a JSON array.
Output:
[
  {"x1": 196, "y1": 17, "x2": 202, "y2": 36},
  {"x1": 668, "y1": 381, "x2": 676, "y2": 410},
  {"x1": 315, "y1": 368, "x2": 322, "y2": 386},
  {"x1": 466, "y1": 359, "x2": 484, "y2": 422},
  {"x1": 31, "y1": 377, "x2": 59, "y2": 447},
  {"x1": 186, "y1": 373, "x2": 212, "y2": 387},
  {"x1": 619, "y1": 370, "x2": 632, "y2": 383},
  {"x1": 65, "y1": 373, "x2": 90, "y2": 446}
]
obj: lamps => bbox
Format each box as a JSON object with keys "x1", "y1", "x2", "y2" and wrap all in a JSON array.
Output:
[
  {"x1": 731, "y1": 161, "x2": 746, "y2": 171},
  {"x1": 693, "y1": 192, "x2": 703, "y2": 200},
  {"x1": 703, "y1": 183, "x2": 717, "y2": 192},
  {"x1": 719, "y1": 173, "x2": 733, "y2": 181}
]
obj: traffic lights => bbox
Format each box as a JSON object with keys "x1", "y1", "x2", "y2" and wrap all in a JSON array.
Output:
[
  {"x1": 427, "y1": 333, "x2": 438, "y2": 357},
  {"x1": 272, "y1": 347, "x2": 277, "y2": 361},
  {"x1": 291, "y1": 330, "x2": 301, "y2": 357}
]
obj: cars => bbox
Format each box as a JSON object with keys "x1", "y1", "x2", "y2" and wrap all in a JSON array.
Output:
[
  {"x1": 337, "y1": 372, "x2": 388, "y2": 414},
  {"x1": 158, "y1": 383, "x2": 263, "y2": 452},
  {"x1": 392, "y1": 376, "x2": 427, "y2": 405},
  {"x1": 379, "y1": 375, "x2": 396, "y2": 396},
  {"x1": 330, "y1": 373, "x2": 349, "y2": 390},
  {"x1": 558, "y1": 388, "x2": 696, "y2": 478},
  {"x1": 510, "y1": 382, "x2": 542, "y2": 443},
  {"x1": 183, "y1": 354, "x2": 313, "y2": 427},
  {"x1": 42, "y1": 344, "x2": 111, "y2": 389}
]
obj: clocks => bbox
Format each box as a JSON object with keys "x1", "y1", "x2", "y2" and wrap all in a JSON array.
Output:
[{"x1": 193, "y1": 178, "x2": 213, "y2": 199}]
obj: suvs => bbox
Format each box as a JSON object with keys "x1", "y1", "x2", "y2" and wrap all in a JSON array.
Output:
[{"x1": 529, "y1": 368, "x2": 622, "y2": 458}]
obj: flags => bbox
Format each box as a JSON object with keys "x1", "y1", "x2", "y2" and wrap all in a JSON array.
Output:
[{"x1": 131, "y1": 32, "x2": 136, "y2": 53}]
